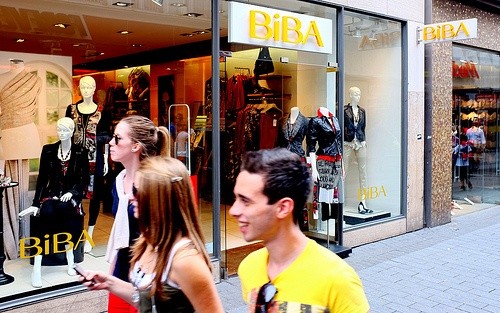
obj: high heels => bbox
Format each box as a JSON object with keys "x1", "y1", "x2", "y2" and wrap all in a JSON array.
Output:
[{"x1": 357, "y1": 201, "x2": 374, "y2": 214}]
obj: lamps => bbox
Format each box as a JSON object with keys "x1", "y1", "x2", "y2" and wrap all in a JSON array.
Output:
[
  {"x1": 368, "y1": 29, "x2": 377, "y2": 41},
  {"x1": 353, "y1": 27, "x2": 361, "y2": 38}
]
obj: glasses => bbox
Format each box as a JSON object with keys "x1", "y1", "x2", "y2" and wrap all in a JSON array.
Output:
[
  {"x1": 112, "y1": 134, "x2": 133, "y2": 145},
  {"x1": 131, "y1": 182, "x2": 140, "y2": 197},
  {"x1": 254, "y1": 283, "x2": 277, "y2": 313}
]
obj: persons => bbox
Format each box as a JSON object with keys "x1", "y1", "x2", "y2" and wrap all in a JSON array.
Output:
[
  {"x1": 18, "y1": 117, "x2": 91, "y2": 288},
  {"x1": 175, "y1": 124, "x2": 188, "y2": 165},
  {"x1": 344, "y1": 86, "x2": 372, "y2": 213},
  {"x1": 126, "y1": 69, "x2": 150, "y2": 109},
  {"x1": 65, "y1": 75, "x2": 110, "y2": 253},
  {"x1": 79, "y1": 155, "x2": 225, "y2": 313},
  {"x1": 229, "y1": 147, "x2": 370, "y2": 313},
  {"x1": 307, "y1": 107, "x2": 345, "y2": 188},
  {"x1": 466, "y1": 118, "x2": 486, "y2": 175},
  {"x1": 278, "y1": 107, "x2": 310, "y2": 162},
  {"x1": 105, "y1": 116, "x2": 170, "y2": 313},
  {"x1": 0, "y1": 59, "x2": 42, "y2": 161},
  {"x1": 453, "y1": 134, "x2": 473, "y2": 192}
]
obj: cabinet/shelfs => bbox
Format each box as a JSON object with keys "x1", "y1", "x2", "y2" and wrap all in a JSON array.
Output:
[{"x1": 245, "y1": 74, "x2": 292, "y2": 101}]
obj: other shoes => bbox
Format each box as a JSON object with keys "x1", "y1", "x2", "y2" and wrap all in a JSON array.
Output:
[{"x1": 466, "y1": 180, "x2": 473, "y2": 189}]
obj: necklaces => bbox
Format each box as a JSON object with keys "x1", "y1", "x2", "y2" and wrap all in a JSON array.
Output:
[
  {"x1": 60, "y1": 143, "x2": 71, "y2": 165},
  {"x1": 131, "y1": 245, "x2": 159, "y2": 303}
]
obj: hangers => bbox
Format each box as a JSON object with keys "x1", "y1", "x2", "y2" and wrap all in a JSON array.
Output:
[
  {"x1": 247, "y1": 98, "x2": 283, "y2": 114},
  {"x1": 229, "y1": 67, "x2": 249, "y2": 79}
]
